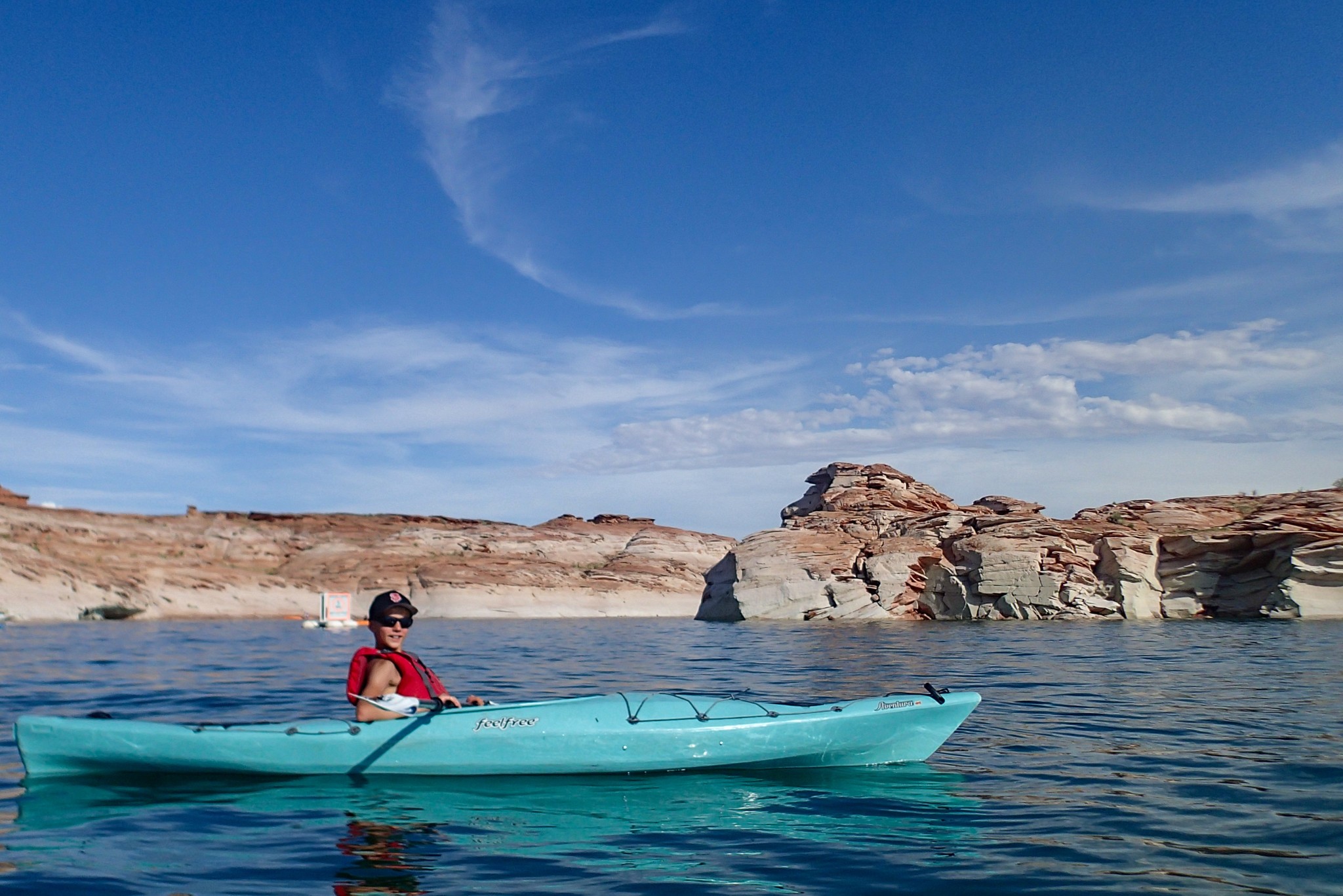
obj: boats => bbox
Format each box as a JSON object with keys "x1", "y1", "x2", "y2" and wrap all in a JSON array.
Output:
[
  {"x1": 10, "y1": 680, "x2": 982, "y2": 784},
  {"x1": 80, "y1": 605, "x2": 145, "y2": 622},
  {"x1": 302, "y1": 614, "x2": 374, "y2": 630}
]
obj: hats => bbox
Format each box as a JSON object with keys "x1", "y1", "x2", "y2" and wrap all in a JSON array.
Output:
[{"x1": 370, "y1": 591, "x2": 418, "y2": 619}]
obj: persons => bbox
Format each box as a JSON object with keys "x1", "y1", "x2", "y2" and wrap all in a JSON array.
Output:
[{"x1": 346, "y1": 589, "x2": 486, "y2": 722}]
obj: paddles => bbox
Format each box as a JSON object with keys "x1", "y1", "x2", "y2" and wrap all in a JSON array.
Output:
[{"x1": 347, "y1": 691, "x2": 500, "y2": 718}]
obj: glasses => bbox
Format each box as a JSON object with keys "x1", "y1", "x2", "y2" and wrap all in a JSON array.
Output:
[{"x1": 370, "y1": 616, "x2": 413, "y2": 628}]
